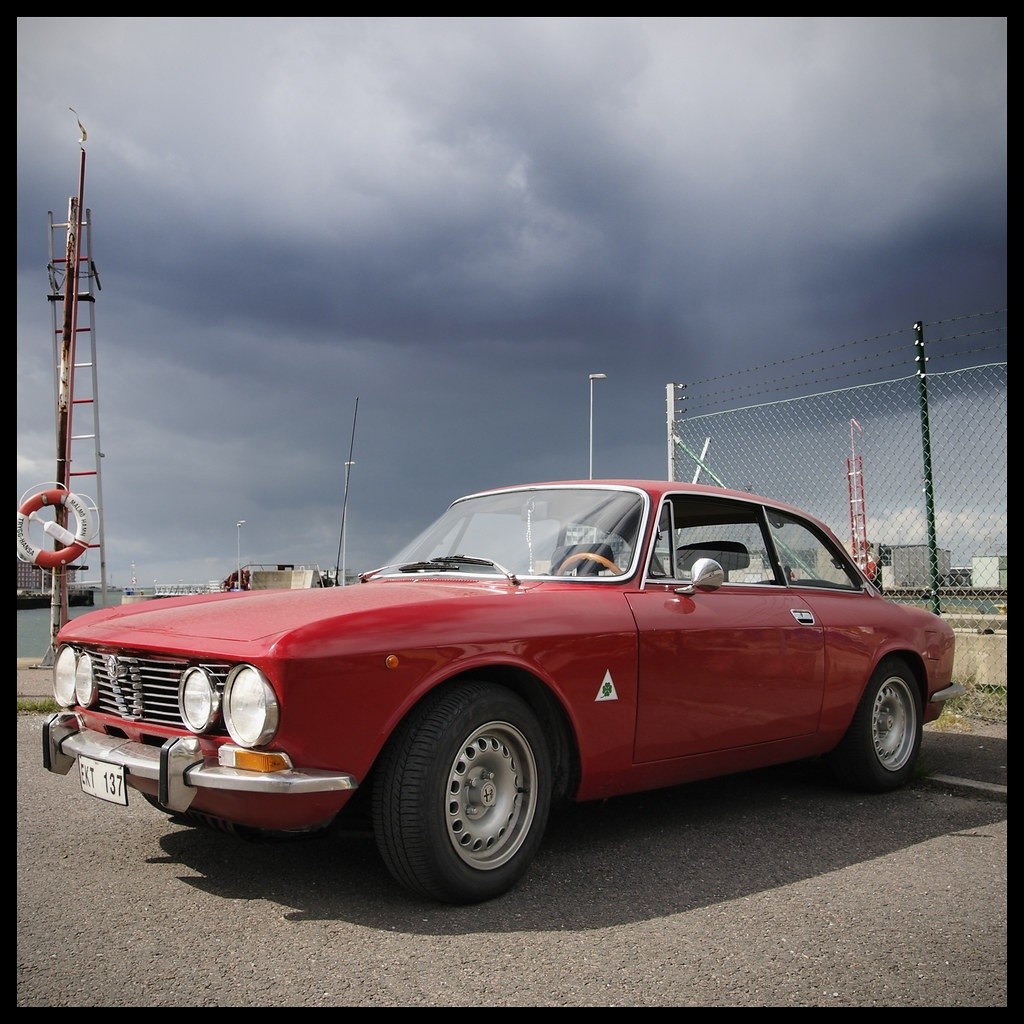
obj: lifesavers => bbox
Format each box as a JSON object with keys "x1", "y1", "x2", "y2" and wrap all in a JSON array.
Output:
[
  {"x1": 17, "y1": 489, "x2": 93, "y2": 571},
  {"x1": 851, "y1": 554, "x2": 876, "y2": 580}
]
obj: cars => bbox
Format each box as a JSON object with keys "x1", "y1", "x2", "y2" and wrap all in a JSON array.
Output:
[{"x1": 41, "y1": 477, "x2": 968, "y2": 905}]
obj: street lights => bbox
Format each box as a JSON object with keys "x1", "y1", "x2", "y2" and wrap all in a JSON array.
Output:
[
  {"x1": 590, "y1": 373, "x2": 607, "y2": 481},
  {"x1": 342, "y1": 461, "x2": 357, "y2": 587},
  {"x1": 236, "y1": 520, "x2": 246, "y2": 588}
]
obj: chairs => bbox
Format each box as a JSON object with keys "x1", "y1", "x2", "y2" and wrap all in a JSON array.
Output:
[
  {"x1": 678, "y1": 541, "x2": 751, "y2": 583},
  {"x1": 552, "y1": 543, "x2": 615, "y2": 575}
]
